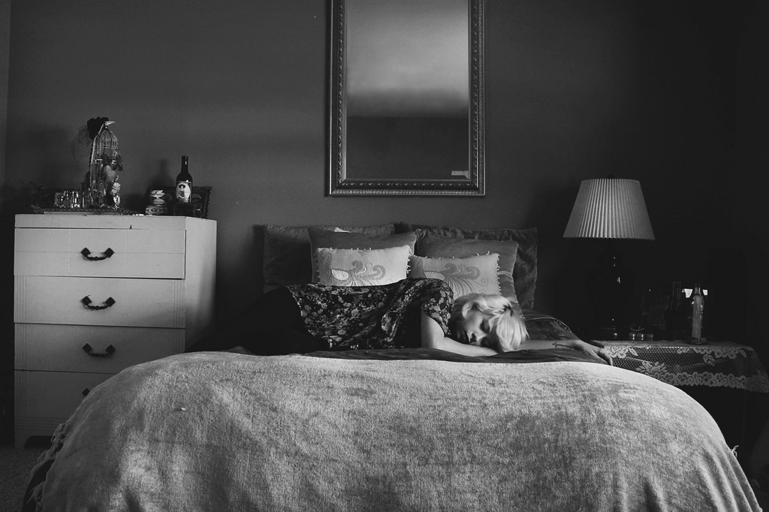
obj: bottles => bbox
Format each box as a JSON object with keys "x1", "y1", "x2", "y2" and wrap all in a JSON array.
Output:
[{"x1": 174, "y1": 154, "x2": 193, "y2": 216}]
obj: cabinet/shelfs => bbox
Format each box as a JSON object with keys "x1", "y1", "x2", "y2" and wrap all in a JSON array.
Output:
[{"x1": 13, "y1": 210, "x2": 221, "y2": 448}]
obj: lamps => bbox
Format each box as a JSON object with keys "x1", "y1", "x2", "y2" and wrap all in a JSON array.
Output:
[{"x1": 564, "y1": 176, "x2": 659, "y2": 338}]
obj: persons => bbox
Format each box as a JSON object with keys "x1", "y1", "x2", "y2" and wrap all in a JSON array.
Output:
[{"x1": 184, "y1": 277, "x2": 615, "y2": 366}]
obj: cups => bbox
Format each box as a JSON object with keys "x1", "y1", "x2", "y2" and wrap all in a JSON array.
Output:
[
  {"x1": 53, "y1": 190, "x2": 102, "y2": 209},
  {"x1": 627, "y1": 326, "x2": 654, "y2": 345}
]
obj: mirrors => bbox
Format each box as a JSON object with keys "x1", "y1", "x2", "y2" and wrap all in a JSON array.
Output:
[{"x1": 328, "y1": 0, "x2": 487, "y2": 198}]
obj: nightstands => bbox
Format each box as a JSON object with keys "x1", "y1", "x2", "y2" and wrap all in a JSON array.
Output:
[{"x1": 590, "y1": 335, "x2": 769, "y2": 468}]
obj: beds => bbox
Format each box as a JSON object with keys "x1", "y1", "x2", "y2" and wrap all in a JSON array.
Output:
[{"x1": 24, "y1": 221, "x2": 766, "y2": 512}]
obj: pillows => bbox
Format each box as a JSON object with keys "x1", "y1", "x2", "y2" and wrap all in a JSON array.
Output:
[
  {"x1": 405, "y1": 252, "x2": 508, "y2": 302},
  {"x1": 302, "y1": 225, "x2": 420, "y2": 253},
  {"x1": 310, "y1": 243, "x2": 408, "y2": 295},
  {"x1": 404, "y1": 224, "x2": 542, "y2": 311},
  {"x1": 416, "y1": 236, "x2": 524, "y2": 308},
  {"x1": 256, "y1": 220, "x2": 403, "y2": 294}
]
